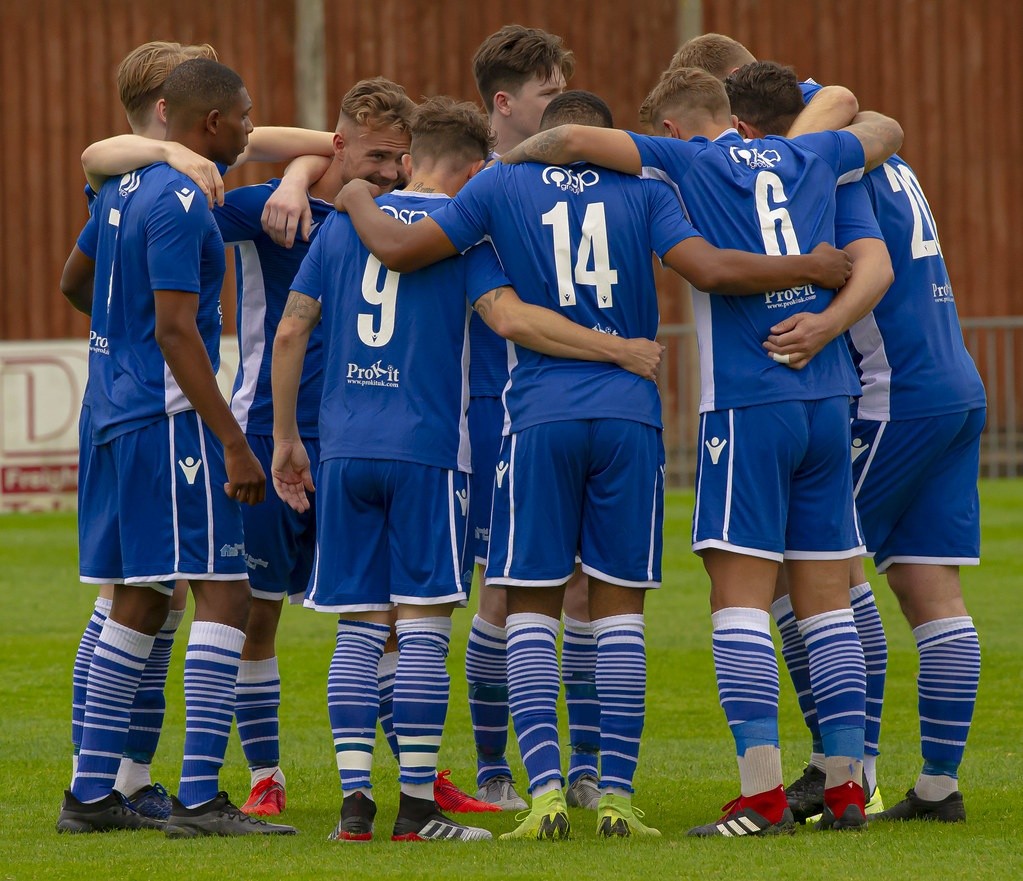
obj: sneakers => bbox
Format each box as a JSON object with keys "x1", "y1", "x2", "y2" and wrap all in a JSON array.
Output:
[
  {"x1": 328, "y1": 790, "x2": 377, "y2": 842},
  {"x1": 595, "y1": 793, "x2": 662, "y2": 839},
  {"x1": 688, "y1": 783, "x2": 796, "y2": 837},
  {"x1": 476, "y1": 775, "x2": 529, "y2": 812},
  {"x1": 498, "y1": 787, "x2": 574, "y2": 839},
  {"x1": 566, "y1": 773, "x2": 602, "y2": 810},
  {"x1": 238, "y1": 769, "x2": 287, "y2": 817},
  {"x1": 866, "y1": 788, "x2": 967, "y2": 825},
  {"x1": 123, "y1": 783, "x2": 174, "y2": 823},
  {"x1": 55, "y1": 789, "x2": 168, "y2": 834},
  {"x1": 164, "y1": 790, "x2": 295, "y2": 839},
  {"x1": 433, "y1": 769, "x2": 504, "y2": 814},
  {"x1": 391, "y1": 792, "x2": 492, "y2": 840},
  {"x1": 784, "y1": 763, "x2": 885, "y2": 832}
]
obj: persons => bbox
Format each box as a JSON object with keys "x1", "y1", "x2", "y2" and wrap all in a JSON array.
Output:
[
  {"x1": 55, "y1": 24, "x2": 989, "y2": 840},
  {"x1": 331, "y1": 92, "x2": 854, "y2": 838}
]
